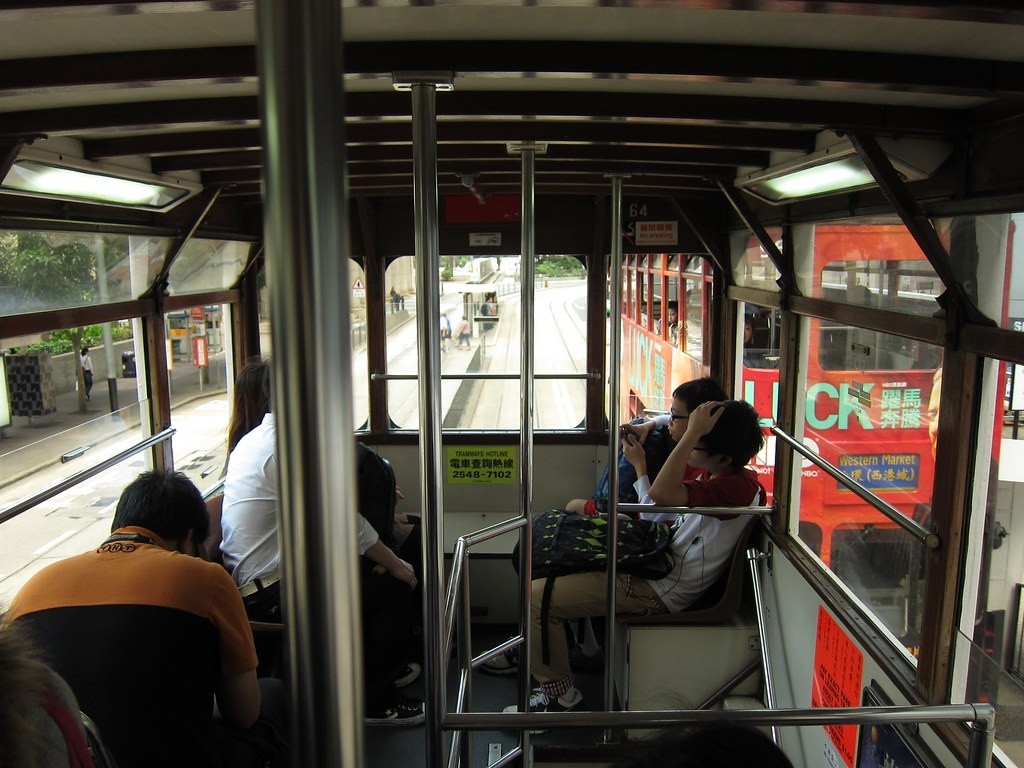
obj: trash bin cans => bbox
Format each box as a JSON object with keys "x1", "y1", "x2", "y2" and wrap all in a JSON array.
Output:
[{"x1": 121, "y1": 350, "x2": 136, "y2": 379}]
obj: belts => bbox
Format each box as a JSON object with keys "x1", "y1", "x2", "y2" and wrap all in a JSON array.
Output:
[{"x1": 238, "y1": 570, "x2": 281, "y2": 597}]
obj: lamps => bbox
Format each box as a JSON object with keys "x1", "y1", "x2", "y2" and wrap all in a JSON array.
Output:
[
  {"x1": 735, "y1": 140, "x2": 931, "y2": 208},
  {"x1": 0, "y1": 143, "x2": 205, "y2": 213}
]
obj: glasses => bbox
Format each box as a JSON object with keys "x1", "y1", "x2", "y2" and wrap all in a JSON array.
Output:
[{"x1": 670, "y1": 407, "x2": 689, "y2": 421}]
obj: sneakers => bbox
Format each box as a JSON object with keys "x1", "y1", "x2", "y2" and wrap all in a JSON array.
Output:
[
  {"x1": 384, "y1": 662, "x2": 421, "y2": 687},
  {"x1": 363, "y1": 696, "x2": 425, "y2": 727},
  {"x1": 503, "y1": 688, "x2": 585, "y2": 735},
  {"x1": 477, "y1": 637, "x2": 520, "y2": 674},
  {"x1": 567, "y1": 643, "x2": 605, "y2": 674}
]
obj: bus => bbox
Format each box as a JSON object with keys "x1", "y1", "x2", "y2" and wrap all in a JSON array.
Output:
[{"x1": 604, "y1": 216, "x2": 1017, "y2": 663}]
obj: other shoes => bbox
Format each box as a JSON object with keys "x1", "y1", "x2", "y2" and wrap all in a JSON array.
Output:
[{"x1": 85, "y1": 394, "x2": 91, "y2": 400}]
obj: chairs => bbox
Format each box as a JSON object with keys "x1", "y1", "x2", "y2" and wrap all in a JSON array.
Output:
[
  {"x1": 613, "y1": 518, "x2": 755, "y2": 629},
  {"x1": 198, "y1": 491, "x2": 287, "y2": 636},
  {"x1": 0, "y1": 647, "x2": 119, "y2": 768}
]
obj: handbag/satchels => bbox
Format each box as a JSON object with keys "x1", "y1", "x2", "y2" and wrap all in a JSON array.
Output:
[
  {"x1": 76, "y1": 367, "x2": 85, "y2": 377},
  {"x1": 75, "y1": 380, "x2": 78, "y2": 391},
  {"x1": 458, "y1": 332, "x2": 465, "y2": 340}
]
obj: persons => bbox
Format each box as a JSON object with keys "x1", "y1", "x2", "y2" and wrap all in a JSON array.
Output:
[
  {"x1": 456, "y1": 317, "x2": 471, "y2": 350},
  {"x1": 480, "y1": 401, "x2": 765, "y2": 736},
  {"x1": 482, "y1": 296, "x2": 497, "y2": 330},
  {"x1": 928, "y1": 368, "x2": 942, "y2": 456},
  {"x1": 80, "y1": 347, "x2": 93, "y2": 400},
  {"x1": 668, "y1": 301, "x2": 677, "y2": 337},
  {"x1": 0, "y1": 469, "x2": 286, "y2": 768},
  {"x1": 390, "y1": 287, "x2": 395, "y2": 298},
  {"x1": 566, "y1": 378, "x2": 731, "y2": 519},
  {"x1": 444, "y1": 314, "x2": 458, "y2": 345},
  {"x1": 0, "y1": 615, "x2": 88, "y2": 768},
  {"x1": 394, "y1": 292, "x2": 400, "y2": 311},
  {"x1": 440, "y1": 313, "x2": 448, "y2": 352},
  {"x1": 743, "y1": 313, "x2": 780, "y2": 369},
  {"x1": 221, "y1": 354, "x2": 427, "y2": 728}
]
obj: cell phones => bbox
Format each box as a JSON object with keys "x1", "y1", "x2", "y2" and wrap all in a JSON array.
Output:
[{"x1": 623, "y1": 428, "x2": 639, "y2": 446}]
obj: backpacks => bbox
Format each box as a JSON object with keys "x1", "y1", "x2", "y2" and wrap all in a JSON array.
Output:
[
  {"x1": 592, "y1": 419, "x2": 678, "y2": 520},
  {"x1": 512, "y1": 509, "x2": 676, "y2": 666}
]
